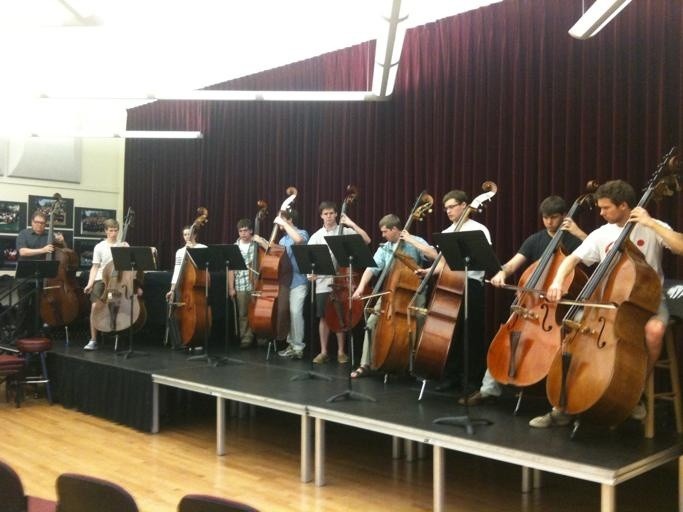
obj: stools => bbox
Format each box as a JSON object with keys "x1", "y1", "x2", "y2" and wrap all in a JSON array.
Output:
[
  {"x1": 1, "y1": 353, "x2": 26, "y2": 408},
  {"x1": 6, "y1": 335, "x2": 54, "y2": 406},
  {"x1": 639, "y1": 316, "x2": 682, "y2": 438}
]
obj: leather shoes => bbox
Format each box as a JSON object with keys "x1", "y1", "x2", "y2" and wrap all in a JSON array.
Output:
[{"x1": 459, "y1": 391, "x2": 497, "y2": 407}]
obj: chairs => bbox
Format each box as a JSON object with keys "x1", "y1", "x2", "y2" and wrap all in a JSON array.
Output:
[
  {"x1": 52, "y1": 473, "x2": 141, "y2": 511},
  {"x1": 174, "y1": 491, "x2": 258, "y2": 511},
  {"x1": 1, "y1": 456, "x2": 56, "y2": 511}
]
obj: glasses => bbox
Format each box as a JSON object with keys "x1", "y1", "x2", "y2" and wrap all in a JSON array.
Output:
[
  {"x1": 34, "y1": 219, "x2": 47, "y2": 225},
  {"x1": 443, "y1": 204, "x2": 461, "y2": 213}
]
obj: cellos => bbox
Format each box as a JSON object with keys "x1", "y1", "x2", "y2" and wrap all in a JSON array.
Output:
[
  {"x1": 369, "y1": 194, "x2": 433, "y2": 371},
  {"x1": 248, "y1": 199, "x2": 267, "y2": 332},
  {"x1": 39, "y1": 194, "x2": 84, "y2": 327},
  {"x1": 92, "y1": 208, "x2": 147, "y2": 335},
  {"x1": 174, "y1": 208, "x2": 211, "y2": 345},
  {"x1": 547, "y1": 145, "x2": 682, "y2": 426},
  {"x1": 413, "y1": 180, "x2": 497, "y2": 381},
  {"x1": 247, "y1": 187, "x2": 298, "y2": 340},
  {"x1": 486, "y1": 178, "x2": 600, "y2": 387},
  {"x1": 324, "y1": 184, "x2": 374, "y2": 333}
]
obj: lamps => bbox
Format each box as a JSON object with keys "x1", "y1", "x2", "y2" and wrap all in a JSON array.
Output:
[
  {"x1": 32, "y1": 86, "x2": 396, "y2": 104},
  {"x1": 561, "y1": 0, "x2": 633, "y2": 44},
  {"x1": 25, "y1": 127, "x2": 206, "y2": 141},
  {"x1": 367, "y1": 0, "x2": 415, "y2": 100}
]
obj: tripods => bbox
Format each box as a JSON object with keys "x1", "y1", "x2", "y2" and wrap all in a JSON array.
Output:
[
  {"x1": 114, "y1": 261, "x2": 150, "y2": 360},
  {"x1": 187, "y1": 262, "x2": 219, "y2": 366},
  {"x1": 433, "y1": 262, "x2": 494, "y2": 435},
  {"x1": 213, "y1": 260, "x2": 247, "y2": 366},
  {"x1": 289, "y1": 262, "x2": 334, "y2": 383},
  {"x1": 326, "y1": 256, "x2": 377, "y2": 403}
]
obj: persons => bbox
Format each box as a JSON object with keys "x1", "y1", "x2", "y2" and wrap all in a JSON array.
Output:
[
  {"x1": 461, "y1": 179, "x2": 683, "y2": 428},
  {"x1": 228, "y1": 204, "x2": 494, "y2": 391},
  {"x1": 84, "y1": 218, "x2": 144, "y2": 351},
  {"x1": 164, "y1": 227, "x2": 213, "y2": 357},
  {"x1": 16, "y1": 212, "x2": 67, "y2": 340}
]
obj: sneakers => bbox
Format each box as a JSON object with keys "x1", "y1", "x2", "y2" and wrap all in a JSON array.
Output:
[
  {"x1": 84, "y1": 341, "x2": 99, "y2": 351},
  {"x1": 240, "y1": 343, "x2": 249, "y2": 351},
  {"x1": 529, "y1": 413, "x2": 570, "y2": 428},
  {"x1": 313, "y1": 354, "x2": 330, "y2": 365},
  {"x1": 338, "y1": 354, "x2": 349, "y2": 364},
  {"x1": 277, "y1": 347, "x2": 304, "y2": 361},
  {"x1": 631, "y1": 401, "x2": 647, "y2": 421}
]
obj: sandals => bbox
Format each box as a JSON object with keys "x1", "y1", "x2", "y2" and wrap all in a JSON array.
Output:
[{"x1": 350, "y1": 364, "x2": 371, "y2": 379}]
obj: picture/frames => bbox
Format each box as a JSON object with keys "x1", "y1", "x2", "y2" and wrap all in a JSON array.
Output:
[
  {"x1": 73, "y1": 236, "x2": 103, "y2": 268},
  {"x1": 25, "y1": 195, "x2": 73, "y2": 229},
  {"x1": 0, "y1": 235, "x2": 19, "y2": 272},
  {"x1": 0, "y1": 198, "x2": 28, "y2": 233},
  {"x1": 73, "y1": 207, "x2": 116, "y2": 239}
]
